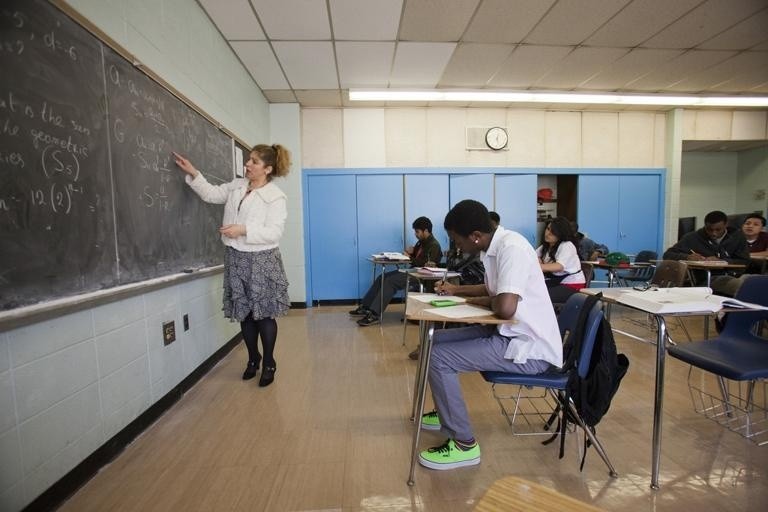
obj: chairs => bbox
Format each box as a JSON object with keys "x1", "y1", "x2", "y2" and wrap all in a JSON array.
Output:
[
  {"x1": 710, "y1": 277, "x2": 733, "y2": 295},
  {"x1": 651, "y1": 261, "x2": 688, "y2": 287},
  {"x1": 480, "y1": 293, "x2": 618, "y2": 479},
  {"x1": 605, "y1": 250, "x2": 659, "y2": 289},
  {"x1": 722, "y1": 279, "x2": 743, "y2": 297},
  {"x1": 666, "y1": 275, "x2": 768, "y2": 446},
  {"x1": 580, "y1": 263, "x2": 593, "y2": 287}
]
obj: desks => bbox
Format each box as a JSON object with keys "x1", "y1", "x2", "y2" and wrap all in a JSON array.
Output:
[
  {"x1": 649, "y1": 259, "x2": 745, "y2": 289},
  {"x1": 561, "y1": 288, "x2": 768, "y2": 492},
  {"x1": 367, "y1": 257, "x2": 412, "y2": 325},
  {"x1": 580, "y1": 262, "x2": 649, "y2": 325},
  {"x1": 398, "y1": 268, "x2": 444, "y2": 346},
  {"x1": 404, "y1": 292, "x2": 518, "y2": 487}
]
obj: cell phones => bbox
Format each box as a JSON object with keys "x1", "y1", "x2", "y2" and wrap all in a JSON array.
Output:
[{"x1": 430, "y1": 299, "x2": 457, "y2": 307}]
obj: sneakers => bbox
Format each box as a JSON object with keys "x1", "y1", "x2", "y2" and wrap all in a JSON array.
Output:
[
  {"x1": 417, "y1": 409, "x2": 442, "y2": 431},
  {"x1": 357, "y1": 311, "x2": 380, "y2": 326},
  {"x1": 349, "y1": 304, "x2": 374, "y2": 317},
  {"x1": 417, "y1": 437, "x2": 481, "y2": 471}
]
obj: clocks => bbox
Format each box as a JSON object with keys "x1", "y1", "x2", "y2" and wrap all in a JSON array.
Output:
[{"x1": 486, "y1": 126, "x2": 509, "y2": 152}]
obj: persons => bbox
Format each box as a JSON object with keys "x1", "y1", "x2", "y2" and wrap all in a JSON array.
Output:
[
  {"x1": 733, "y1": 217, "x2": 763, "y2": 254},
  {"x1": 662, "y1": 211, "x2": 751, "y2": 332},
  {"x1": 349, "y1": 218, "x2": 443, "y2": 326},
  {"x1": 568, "y1": 224, "x2": 608, "y2": 282},
  {"x1": 526, "y1": 219, "x2": 586, "y2": 303},
  {"x1": 414, "y1": 200, "x2": 564, "y2": 471},
  {"x1": 174, "y1": 144, "x2": 291, "y2": 388}
]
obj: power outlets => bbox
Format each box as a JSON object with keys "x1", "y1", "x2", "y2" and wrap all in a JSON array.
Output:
[{"x1": 163, "y1": 320, "x2": 176, "y2": 346}]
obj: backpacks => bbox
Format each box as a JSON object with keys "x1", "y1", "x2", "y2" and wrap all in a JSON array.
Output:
[{"x1": 540, "y1": 293, "x2": 630, "y2": 477}]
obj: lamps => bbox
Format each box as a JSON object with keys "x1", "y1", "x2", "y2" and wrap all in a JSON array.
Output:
[{"x1": 347, "y1": 88, "x2": 767, "y2": 109}]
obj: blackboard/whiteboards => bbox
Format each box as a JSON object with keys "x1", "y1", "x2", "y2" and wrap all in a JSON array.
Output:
[{"x1": 0, "y1": 0, "x2": 254, "y2": 331}]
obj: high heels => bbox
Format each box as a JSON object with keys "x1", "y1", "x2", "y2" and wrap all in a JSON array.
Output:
[
  {"x1": 242, "y1": 352, "x2": 262, "y2": 380},
  {"x1": 259, "y1": 358, "x2": 276, "y2": 386}
]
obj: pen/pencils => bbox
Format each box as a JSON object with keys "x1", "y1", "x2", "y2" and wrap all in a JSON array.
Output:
[
  {"x1": 440, "y1": 271, "x2": 447, "y2": 296},
  {"x1": 690, "y1": 249, "x2": 695, "y2": 254}
]
